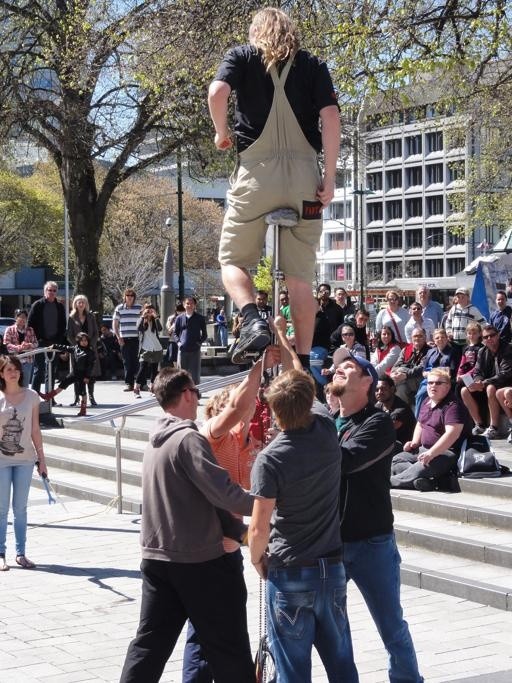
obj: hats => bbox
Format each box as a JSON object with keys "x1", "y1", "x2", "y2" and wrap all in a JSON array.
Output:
[
  {"x1": 455, "y1": 287, "x2": 469, "y2": 297},
  {"x1": 347, "y1": 349, "x2": 379, "y2": 398}
]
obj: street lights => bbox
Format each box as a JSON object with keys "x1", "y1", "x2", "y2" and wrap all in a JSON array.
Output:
[{"x1": 347, "y1": 184, "x2": 374, "y2": 309}]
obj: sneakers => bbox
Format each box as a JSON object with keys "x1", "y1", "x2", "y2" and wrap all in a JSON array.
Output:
[
  {"x1": 471, "y1": 423, "x2": 512, "y2": 443},
  {"x1": 123, "y1": 385, "x2": 156, "y2": 398}
]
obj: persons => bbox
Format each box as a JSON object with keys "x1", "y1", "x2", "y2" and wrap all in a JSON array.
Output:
[
  {"x1": 0, "y1": 281, "x2": 298, "y2": 418},
  {"x1": 207, "y1": 6, "x2": 341, "y2": 378},
  {"x1": 304, "y1": 281, "x2": 512, "y2": 491},
  {"x1": 1, "y1": 355, "x2": 47, "y2": 570},
  {"x1": 120, "y1": 355, "x2": 426, "y2": 682}
]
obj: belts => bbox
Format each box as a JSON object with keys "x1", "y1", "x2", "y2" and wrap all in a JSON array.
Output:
[{"x1": 283, "y1": 555, "x2": 341, "y2": 566}]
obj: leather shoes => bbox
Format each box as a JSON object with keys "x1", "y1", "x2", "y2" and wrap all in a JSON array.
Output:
[
  {"x1": 412, "y1": 478, "x2": 435, "y2": 492},
  {"x1": 232, "y1": 318, "x2": 271, "y2": 365}
]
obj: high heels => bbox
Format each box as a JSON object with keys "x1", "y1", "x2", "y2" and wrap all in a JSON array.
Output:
[
  {"x1": 90, "y1": 400, "x2": 97, "y2": 407},
  {"x1": 71, "y1": 399, "x2": 80, "y2": 405}
]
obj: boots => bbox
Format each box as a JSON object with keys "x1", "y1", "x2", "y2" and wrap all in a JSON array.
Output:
[
  {"x1": 77, "y1": 401, "x2": 86, "y2": 416},
  {"x1": 37, "y1": 390, "x2": 57, "y2": 402}
]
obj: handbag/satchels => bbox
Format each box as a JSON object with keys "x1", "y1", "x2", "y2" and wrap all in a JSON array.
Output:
[
  {"x1": 253, "y1": 643, "x2": 277, "y2": 683},
  {"x1": 457, "y1": 434, "x2": 510, "y2": 478}
]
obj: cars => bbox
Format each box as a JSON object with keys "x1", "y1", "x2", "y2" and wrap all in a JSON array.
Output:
[
  {"x1": 0, "y1": 316, "x2": 17, "y2": 335},
  {"x1": 102, "y1": 314, "x2": 112, "y2": 320}
]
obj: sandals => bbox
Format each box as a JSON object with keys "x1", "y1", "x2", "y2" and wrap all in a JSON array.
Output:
[
  {"x1": 0, "y1": 556, "x2": 9, "y2": 571},
  {"x1": 15, "y1": 555, "x2": 36, "y2": 568}
]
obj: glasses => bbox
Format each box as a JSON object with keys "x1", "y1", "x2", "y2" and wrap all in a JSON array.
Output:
[
  {"x1": 182, "y1": 387, "x2": 202, "y2": 400},
  {"x1": 428, "y1": 381, "x2": 445, "y2": 387},
  {"x1": 482, "y1": 333, "x2": 498, "y2": 339}
]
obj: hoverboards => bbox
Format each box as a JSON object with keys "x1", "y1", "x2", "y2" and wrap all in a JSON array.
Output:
[{"x1": 253, "y1": 206, "x2": 301, "y2": 683}]
